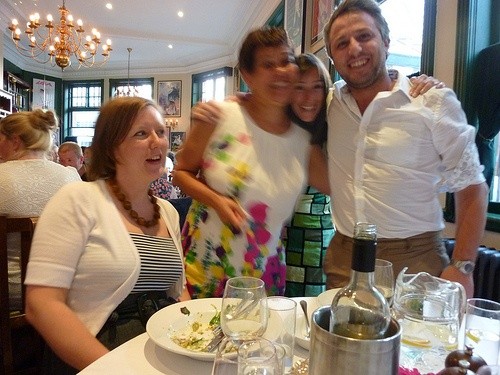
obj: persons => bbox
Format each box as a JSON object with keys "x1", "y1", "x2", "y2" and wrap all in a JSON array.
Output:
[
  {"x1": 190, "y1": 52, "x2": 445, "y2": 301},
  {"x1": 0, "y1": 108, "x2": 83, "y2": 312},
  {"x1": 223, "y1": 0, "x2": 489, "y2": 313},
  {"x1": 57, "y1": 141, "x2": 89, "y2": 182},
  {"x1": 23, "y1": 96, "x2": 193, "y2": 375},
  {"x1": 171, "y1": 23, "x2": 332, "y2": 299}
]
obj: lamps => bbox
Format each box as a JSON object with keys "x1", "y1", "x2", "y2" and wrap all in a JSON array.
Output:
[
  {"x1": 8, "y1": 0, "x2": 113, "y2": 72},
  {"x1": 114, "y1": 48, "x2": 140, "y2": 97}
]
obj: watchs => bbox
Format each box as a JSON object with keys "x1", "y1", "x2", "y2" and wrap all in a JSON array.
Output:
[{"x1": 447, "y1": 259, "x2": 475, "y2": 274}]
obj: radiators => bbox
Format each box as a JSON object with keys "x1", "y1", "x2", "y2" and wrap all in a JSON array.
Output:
[{"x1": 443, "y1": 238, "x2": 500, "y2": 319}]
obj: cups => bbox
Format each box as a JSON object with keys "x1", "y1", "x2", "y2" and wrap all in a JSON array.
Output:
[
  {"x1": 259, "y1": 296, "x2": 297, "y2": 375},
  {"x1": 354, "y1": 259, "x2": 394, "y2": 310},
  {"x1": 391, "y1": 294, "x2": 459, "y2": 375},
  {"x1": 463, "y1": 298, "x2": 500, "y2": 365},
  {"x1": 394, "y1": 268, "x2": 466, "y2": 330},
  {"x1": 211, "y1": 335, "x2": 287, "y2": 375},
  {"x1": 220, "y1": 276, "x2": 269, "y2": 351}
]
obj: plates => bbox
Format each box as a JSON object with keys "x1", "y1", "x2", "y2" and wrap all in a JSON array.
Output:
[
  {"x1": 146, "y1": 298, "x2": 283, "y2": 362},
  {"x1": 315, "y1": 288, "x2": 353, "y2": 305}
]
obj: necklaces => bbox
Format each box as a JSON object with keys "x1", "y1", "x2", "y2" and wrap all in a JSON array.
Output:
[{"x1": 106, "y1": 177, "x2": 161, "y2": 228}]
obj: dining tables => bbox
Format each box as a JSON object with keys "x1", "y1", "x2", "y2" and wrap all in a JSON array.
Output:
[{"x1": 75, "y1": 296, "x2": 500, "y2": 375}]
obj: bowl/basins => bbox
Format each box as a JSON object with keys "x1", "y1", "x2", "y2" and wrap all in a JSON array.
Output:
[{"x1": 284, "y1": 313, "x2": 310, "y2": 351}]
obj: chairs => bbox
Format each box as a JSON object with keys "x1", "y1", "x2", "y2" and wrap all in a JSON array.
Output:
[{"x1": 0, "y1": 215, "x2": 34, "y2": 375}]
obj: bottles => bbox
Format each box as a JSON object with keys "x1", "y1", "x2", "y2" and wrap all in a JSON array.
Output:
[{"x1": 329, "y1": 221, "x2": 391, "y2": 340}]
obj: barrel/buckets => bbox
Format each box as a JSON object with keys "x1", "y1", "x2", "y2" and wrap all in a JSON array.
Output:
[{"x1": 309, "y1": 304, "x2": 402, "y2": 375}]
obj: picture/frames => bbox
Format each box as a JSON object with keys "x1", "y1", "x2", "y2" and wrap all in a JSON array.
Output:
[
  {"x1": 157, "y1": 80, "x2": 182, "y2": 118},
  {"x1": 301, "y1": 0, "x2": 340, "y2": 55},
  {"x1": 165, "y1": 126, "x2": 186, "y2": 152}
]
